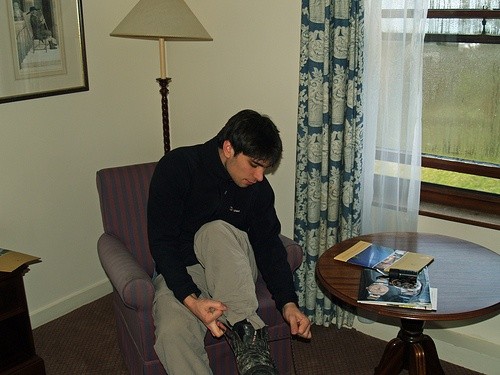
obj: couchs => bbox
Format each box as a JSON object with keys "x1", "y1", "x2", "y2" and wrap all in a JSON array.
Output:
[{"x1": 97, "y1": 162, "x2": 303, "y2": 375}]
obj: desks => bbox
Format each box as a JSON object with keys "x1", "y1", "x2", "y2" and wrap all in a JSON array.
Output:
[
  {"x1": 0, "y1": 265, "x2": 45, "y2": 375},
  {"x1": 315, "y1": 232, "x2": 500, "y2": 375}
]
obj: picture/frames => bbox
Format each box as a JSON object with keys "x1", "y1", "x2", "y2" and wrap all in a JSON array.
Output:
[{"x1": 0, "y1": 0, "x2": 89, "y2": 104}]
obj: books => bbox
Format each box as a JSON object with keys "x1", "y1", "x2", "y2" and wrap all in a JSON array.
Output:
[
  {"x1": 0, "y1": 247, "x2": 42, "y2": 273},
  {"x1": 333, "y1": 239, "x2": 434, "y2": 275},
  {"x1": 357, "y1": 265, "x2": 433, "y2": 307}
]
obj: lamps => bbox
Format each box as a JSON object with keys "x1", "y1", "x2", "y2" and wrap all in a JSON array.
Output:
[{"x1": 110, "y1": 0, "x2": 213, "y2": 157}]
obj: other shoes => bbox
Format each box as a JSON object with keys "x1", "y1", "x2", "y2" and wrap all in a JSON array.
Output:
[{"x1": 215, "y1": 319, "x2": 279, "y2": 375}]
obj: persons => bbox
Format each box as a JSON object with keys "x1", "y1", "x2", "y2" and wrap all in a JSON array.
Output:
[
  {"x1": 26, "y1": 5, "x2": 58, "y2": 50},
  {"x1": 147, "y1": 108, "x2": 313, "y2": 375}
]
